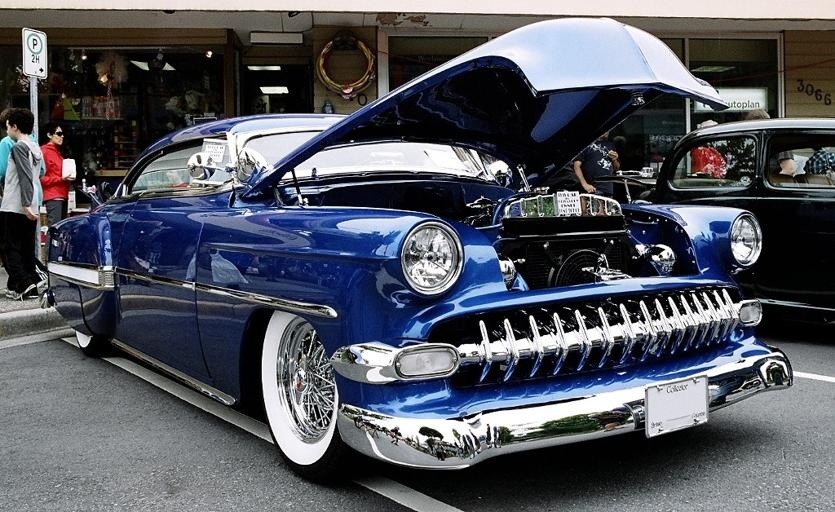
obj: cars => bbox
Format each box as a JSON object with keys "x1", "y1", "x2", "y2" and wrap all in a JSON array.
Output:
[
  {"x1": 637, "y1": 114, "x2": 834, "y2": 338},
  {"x1": 639, "y1": 166, "x2": 657, "y2": 178},
  {"x1": 41, "y1": 17, "x2": 797, "y2": 481}
]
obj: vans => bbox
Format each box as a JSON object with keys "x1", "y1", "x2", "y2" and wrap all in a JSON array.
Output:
[{"x1": 616, "y1": 166, "x2": 642, "y2": 178}]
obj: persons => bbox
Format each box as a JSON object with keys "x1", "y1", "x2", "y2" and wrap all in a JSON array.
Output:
[
  {"x1": 612, "y1": 135, "x2": 635, "y2": 172},
  {"x1": 341, "y1": 406, "x2": 504, "y2": 461},
  {"x1": 37, "y1": 122, "x2": 77, "y2": 254},
  {"x1": 803, "y1": 145, "x2": 835, "y2": 174},
  {"x1": 574, "y1": 132, "x2": 622, "y2": 200},
  {"x1": 742, "y1": 109, "x2": 796, "y2": 176},
  {"x1": 689, "y1": 120, "x2": 726, "y2": 179}
]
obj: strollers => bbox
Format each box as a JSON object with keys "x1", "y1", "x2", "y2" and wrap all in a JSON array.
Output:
[{"x1": 80, "y1": 181, "x2": 114, "y2": 215}]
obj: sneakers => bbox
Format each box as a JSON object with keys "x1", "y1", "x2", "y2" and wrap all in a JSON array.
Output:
[{"x1": 5, "y1": 284, "x2": 36, "y2": 300}]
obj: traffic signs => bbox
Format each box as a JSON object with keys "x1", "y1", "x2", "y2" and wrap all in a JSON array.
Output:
[{"x1": 20, "y1": 27, "x2": 49, "y2": 81}]
